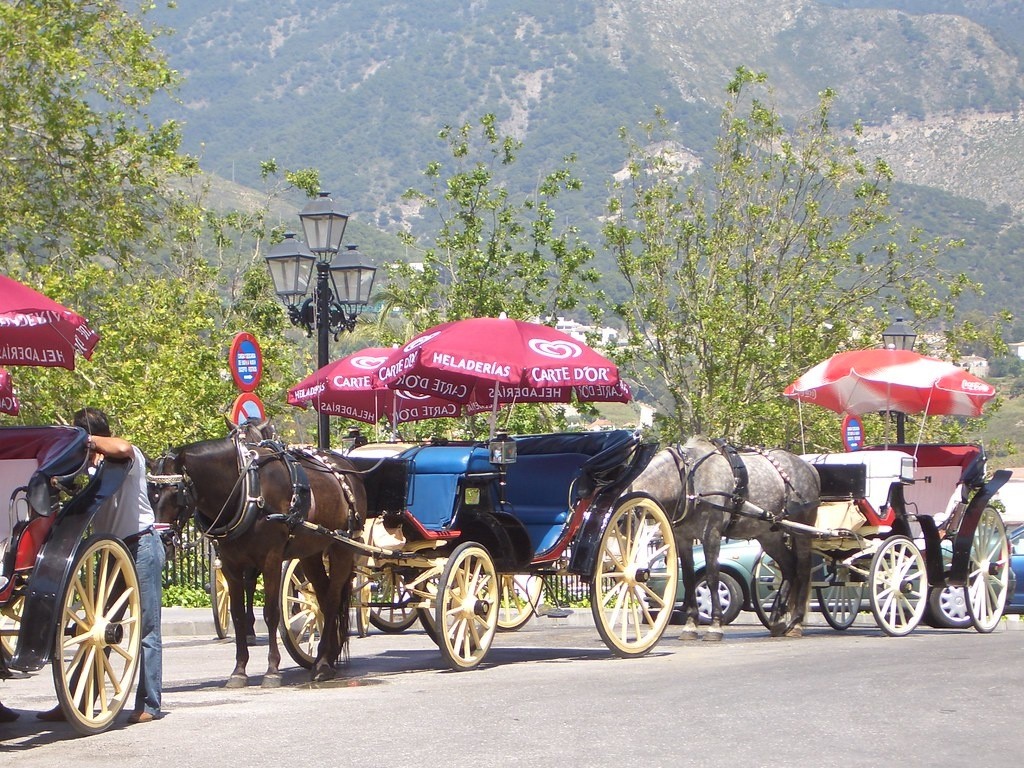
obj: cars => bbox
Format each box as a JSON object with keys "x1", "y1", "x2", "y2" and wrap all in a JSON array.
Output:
[{"x1": 643, "y1": 521, "x2": 1024, "y2": 630}]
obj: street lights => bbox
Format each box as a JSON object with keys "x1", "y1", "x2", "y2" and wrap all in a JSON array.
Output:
[
  {"x1": 264, "y1": 191, "x2": 377, "y2": 452},
  {"x1": 881, "y1": 316, "x2": 918, "y2": 445}
]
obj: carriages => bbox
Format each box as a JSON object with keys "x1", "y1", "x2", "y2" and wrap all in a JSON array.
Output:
[
  {"x1": 613, "y1": 343, "x2": 1012, "y2": 641},
  {"x1": 136, "y1": 311, "x2": 681, "y2": 691}
]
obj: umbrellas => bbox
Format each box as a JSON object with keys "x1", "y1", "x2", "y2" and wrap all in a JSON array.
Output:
[
  {"x1": 287, "y1": 342, "x2": 502, "y2": 443},
  {"x1": 375, "y1": 312, "x2": 634, "y2": 447},
  {"x1": 0, "y1": 274, "x2": 98, "y2": 419},
  {"x1": 782, "y1": 348, "x2": 995, "y2": 458}
]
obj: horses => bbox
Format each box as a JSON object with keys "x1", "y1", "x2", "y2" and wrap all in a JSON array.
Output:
[
  {"x1": 612, "y1": 432, "x2": 822, "y2": 642},
  {"x1": 137, "y1": 414, "x2": 368, "y2": 689}
]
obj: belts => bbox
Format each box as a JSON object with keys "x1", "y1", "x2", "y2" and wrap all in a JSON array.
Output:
[{"x1": 124, "y1": 526, "x2": 155, "y2": 545}]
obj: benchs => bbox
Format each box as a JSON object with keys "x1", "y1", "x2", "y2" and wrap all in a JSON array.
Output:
[
  {"x1": 798, "y1": 451, "x2": 913, "y2": 515},
  {"x1": 346, "y1": 438, "x2": 496, "y2": 530},
  {"x1": 904, "y1": 466, "x2": 963, "y2": 550},
  {"x1": 497, "y1": 452, "x2": 591, "y2": 555},
  {"x1": 0, "y1": 459, "x2": 61, "y2": 603}
]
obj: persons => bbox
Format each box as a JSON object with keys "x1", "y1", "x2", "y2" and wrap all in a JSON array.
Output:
[{"x1": 34, "y1": 410, "x2": 164, "y2": 724}]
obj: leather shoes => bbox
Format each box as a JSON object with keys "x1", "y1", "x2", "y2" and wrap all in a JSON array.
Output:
[
  {"x1": 37, "y1": 703, "x2": 68, "y2": 721},
  {"x1": 127, "y1": 710, "x2": 160, "y2": 723}
]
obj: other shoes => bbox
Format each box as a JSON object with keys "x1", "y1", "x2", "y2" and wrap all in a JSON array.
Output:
[{"x1": 0, "y1": 704, "x2": 20, "y2": 721}]
